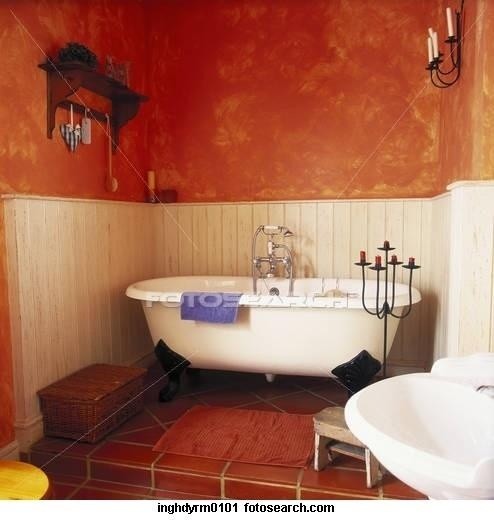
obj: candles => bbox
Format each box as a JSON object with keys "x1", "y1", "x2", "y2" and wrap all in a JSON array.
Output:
[
  {"x1": 424, "y1": 6, "x2": 455, "y2": 65},
  {"x1": 351, "y1": 240, "x2": 418, "y2": 268}
]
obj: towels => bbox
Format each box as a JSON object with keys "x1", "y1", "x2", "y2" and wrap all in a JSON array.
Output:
[{"x1": 179, "y1": 290, "x2": 245, "y2": 325}]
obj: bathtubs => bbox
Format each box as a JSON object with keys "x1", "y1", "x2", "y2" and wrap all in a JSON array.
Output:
[{"x1": 120, "y1": 275, "x2": 418, "y2": 382}]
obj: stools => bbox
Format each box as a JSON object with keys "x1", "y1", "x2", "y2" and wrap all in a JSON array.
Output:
[{"x1": 312, "y1": 405, "x2": 389, "y2": 489}]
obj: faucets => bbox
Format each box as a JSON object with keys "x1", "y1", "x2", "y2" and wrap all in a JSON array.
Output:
[
  {"x1": 475, "y1": 383, "x2": 494, "y2": 396},
  {"x1": 255, "y1": 238, "x2": 290, "y2": 278}
]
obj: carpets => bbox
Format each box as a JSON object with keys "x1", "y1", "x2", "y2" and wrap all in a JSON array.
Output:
[{"x1": 151, "y1": 402, "x2": 320, "y2": 469}]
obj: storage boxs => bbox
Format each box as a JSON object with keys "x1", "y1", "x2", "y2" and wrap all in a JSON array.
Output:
[{"x1": 37, "y1": 360, "x2": 147, "y2": 444}]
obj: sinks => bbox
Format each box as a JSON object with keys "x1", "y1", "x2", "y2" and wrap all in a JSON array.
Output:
[{"x1": 343, "y1": 354, "x2": 494, "y2": 500}]
